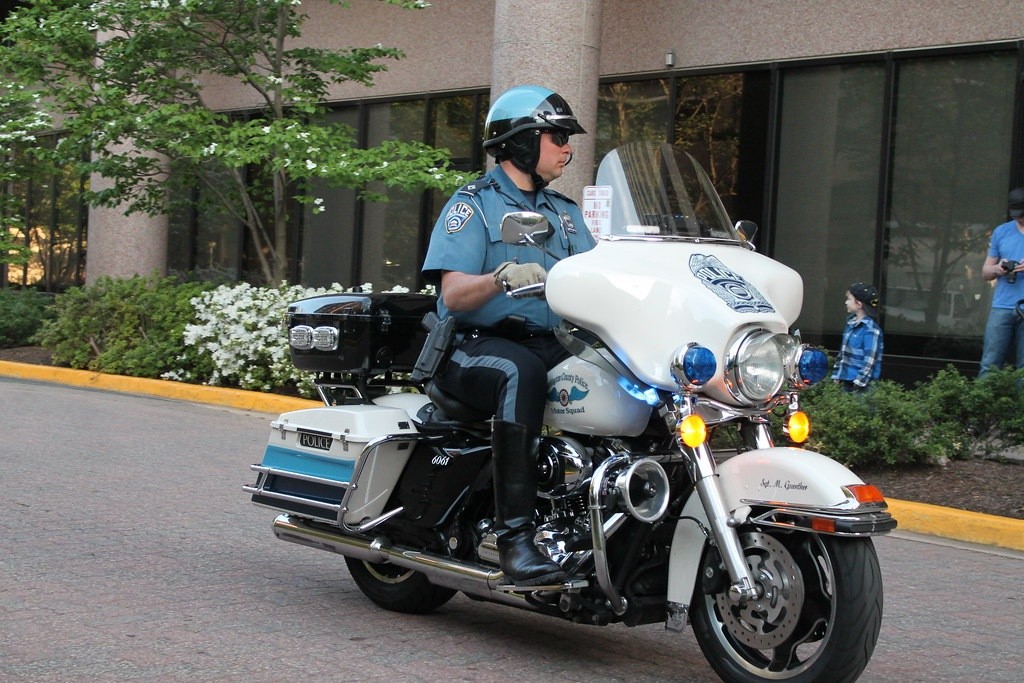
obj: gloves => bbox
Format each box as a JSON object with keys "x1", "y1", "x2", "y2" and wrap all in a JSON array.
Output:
[{"x1": 492, "y1": 262, "x2": 547, "y2": 300}]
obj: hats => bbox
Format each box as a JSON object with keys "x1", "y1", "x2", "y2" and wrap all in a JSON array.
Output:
[
  {"x1": 1007, "y1": 189, "x2": 1024, "y2": 216},
  {"x1": 849, "y1": 282, "x2": 879, "y2": 319}
]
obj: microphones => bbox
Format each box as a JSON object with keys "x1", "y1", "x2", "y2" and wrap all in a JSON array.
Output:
[{"x1": 564, "y1": 147, "x2": 574, "y2": 166}]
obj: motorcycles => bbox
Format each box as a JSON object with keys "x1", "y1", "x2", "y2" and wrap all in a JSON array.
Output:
[{"x1": 239, "y1": 139, "x2": 899, "y2": 683}]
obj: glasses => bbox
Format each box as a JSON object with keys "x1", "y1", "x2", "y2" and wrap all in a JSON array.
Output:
[{"x1": 551, "y1": 132, "x2": 569, "y2": 147}]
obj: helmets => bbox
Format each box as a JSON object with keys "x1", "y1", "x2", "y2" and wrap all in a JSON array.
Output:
[{"x1": 483, "y1": 84, "x2": 587, "y2": 157}]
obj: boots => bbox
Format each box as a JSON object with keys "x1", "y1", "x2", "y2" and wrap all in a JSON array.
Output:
[{"x1": 491, "y1": 412, "x2": 564, "y2": 587}]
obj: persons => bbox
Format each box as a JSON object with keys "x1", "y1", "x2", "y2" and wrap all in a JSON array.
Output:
[
  {"x1": 421, "y1": 85, "x2": 597, "y2": 591},
  {"x1": 967, "y1": 187, "x2": 1024, "y2": 435},
  {"x1": 831, "y1": 282, "x2": 884, "y2": 394}
]
